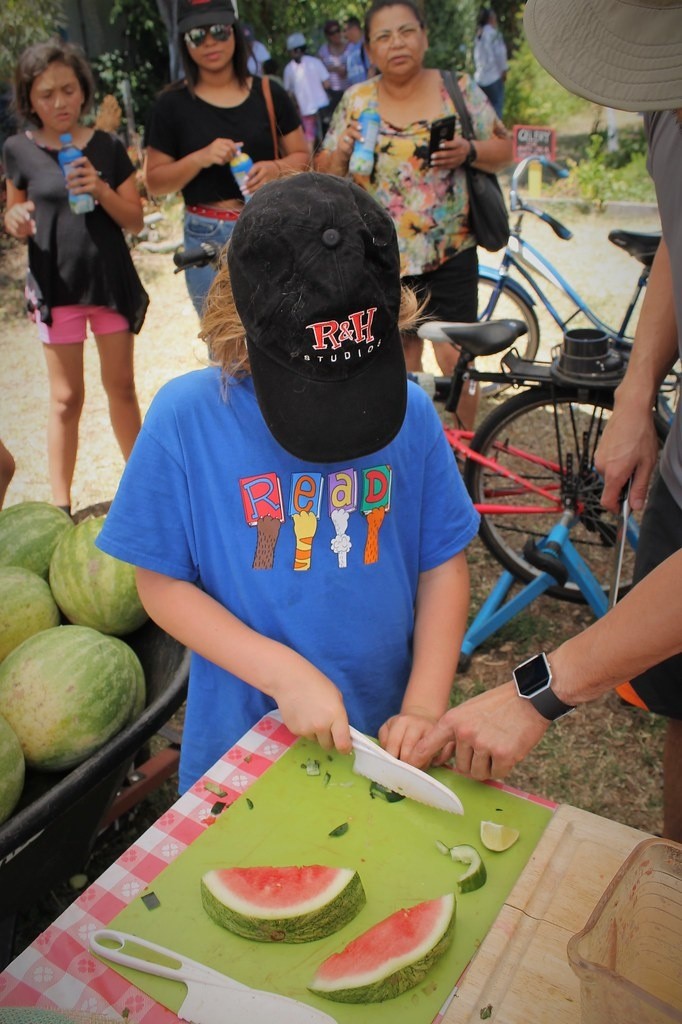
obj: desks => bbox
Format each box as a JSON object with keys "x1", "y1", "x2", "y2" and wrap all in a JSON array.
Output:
[{"x1": 0, "y1": 706, "x2": 682, "y2": 1024}]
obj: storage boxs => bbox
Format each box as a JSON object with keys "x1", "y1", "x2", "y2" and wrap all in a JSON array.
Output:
[{"x1": 565, "y1": 836, "x2": 682, "y2": 1024}]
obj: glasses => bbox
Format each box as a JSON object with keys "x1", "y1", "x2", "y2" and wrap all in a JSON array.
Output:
[
  {"x1": 184, "y1": 24, "x2": 231, "y2": 46},
  {"x1": 330, "y1": 29, "x2": 340, "y2": 35}
]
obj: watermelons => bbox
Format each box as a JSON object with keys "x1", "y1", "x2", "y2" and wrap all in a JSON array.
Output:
[
  {"x1": 0, "y1": 499, "x2": 151, "y2": 827},
  {"x1": 200, "y1": 864, "x2": 367, "y2": 943},
  {"x1": 305, "y1": 892, "x2": 457, "y2": 1004}
]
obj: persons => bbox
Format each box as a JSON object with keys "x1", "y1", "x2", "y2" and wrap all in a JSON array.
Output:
[
  {"x1": 239, "y1": 16, "x2": 377, "y2": 152},
  {"x1": 314, "y1": 0, "x2": 513, "y2": 449},
  {"x1": 3, "y1": 44, "x2": 151, "y2": 515},
  {"x1": 407, "y1": 0, "x2": 682, "y2": 845},
  {"x1": 93, "y1": 169, "x2": 482, "y2": 795},
  {"x1": 140, "y1": 0, "x2": 311, "y2": 364},
  {"x1": 473, "y1": 9, "x2": 510, "y2": 120}
]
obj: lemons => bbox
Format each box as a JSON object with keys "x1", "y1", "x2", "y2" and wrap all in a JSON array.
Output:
[{"x1": 481, "y1": 820, "x2": 519, "y2": 852}]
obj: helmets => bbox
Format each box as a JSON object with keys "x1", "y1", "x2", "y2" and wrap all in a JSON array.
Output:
[{"x1": 286, "y1": 32, "x2": 306, "y2": 50}]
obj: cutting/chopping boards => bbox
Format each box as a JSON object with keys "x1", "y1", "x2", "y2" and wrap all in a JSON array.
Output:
[{"x1": 89, "y1": 728, "x2": 554, "y2": 1024}]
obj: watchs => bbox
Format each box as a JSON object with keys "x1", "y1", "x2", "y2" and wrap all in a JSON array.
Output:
[
  {"x1": 460, "y1": 139, "x2": 477, "y2": 169},
  {"x1": 512, "y1": 653, "x2": 578, "y2": 722}
]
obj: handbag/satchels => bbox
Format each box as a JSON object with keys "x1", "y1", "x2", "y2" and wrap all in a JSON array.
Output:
[{"x1": 441, "y1": 67, "x2": 510, "y2": 253}]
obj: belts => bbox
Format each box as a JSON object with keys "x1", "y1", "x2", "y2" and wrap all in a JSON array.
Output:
[{"x1": 187, "y1": 205, "x2": 240, "y2": 221}]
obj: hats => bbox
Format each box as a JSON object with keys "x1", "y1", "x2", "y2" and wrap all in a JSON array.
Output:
[
  {"x1": 176, "y1": 0, "x2": 235, "y2": 33},
  {"x1": 228, "y1": 171, "x2": 408, "y2": 462},
  {"x1": 522, "y1": 0, "x2": 682, "y2": 112}
]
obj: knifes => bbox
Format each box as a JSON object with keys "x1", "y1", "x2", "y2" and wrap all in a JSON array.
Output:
[
  {"x1": 348, "y1": 726, "x2": 465, "y2": 816},
  {"x1": 608, "y1": 476, "x2": 633, "y2": 614},
  {"x1": 89, "y1": 929, "x2": 338, "y2": 1024}
]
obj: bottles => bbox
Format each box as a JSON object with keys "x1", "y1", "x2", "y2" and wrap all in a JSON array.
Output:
[
  {"x1": 57, "y1": 133, "x2": 94, "y2": 215},
  {"x1": 230, "y1": 146, "x2": 256, "y2": 206},
  {"x1": 348, "y1": 101, "x2": 381, "y2": 177}
]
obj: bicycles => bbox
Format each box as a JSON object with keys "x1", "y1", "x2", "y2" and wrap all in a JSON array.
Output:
[
  {"x1": 420, "y1": 149, "x2": 682, "y2": 447},
  {"x1": 171, "y1": 241, "x2": 674, "y2": 606}
]
